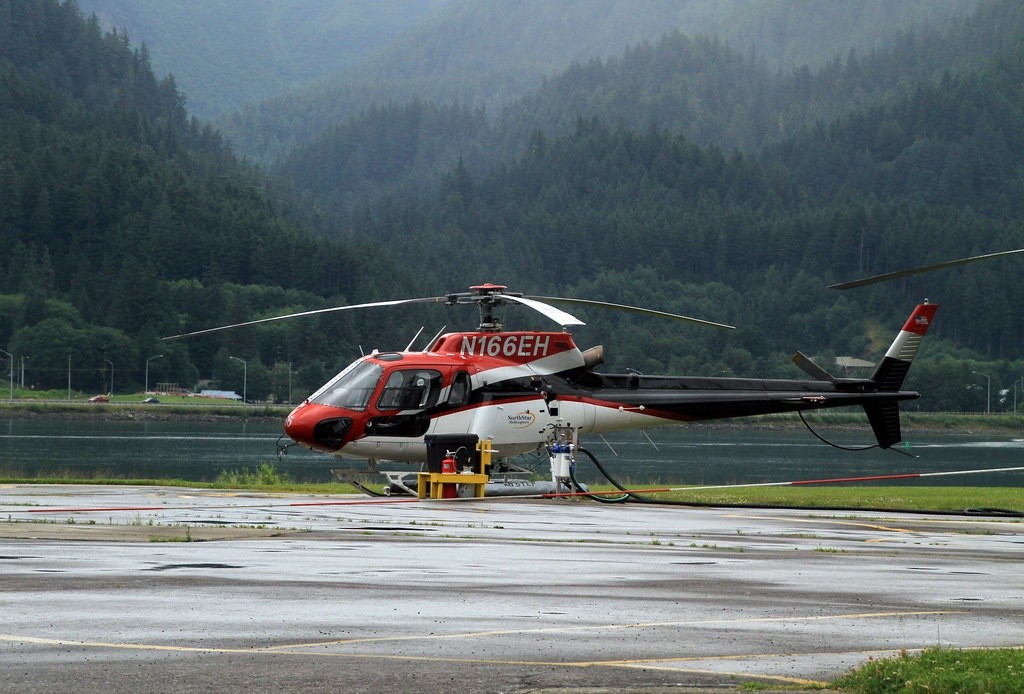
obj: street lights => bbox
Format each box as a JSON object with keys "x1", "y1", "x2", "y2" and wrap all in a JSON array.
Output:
[
  {"x1": 709, "y1": 371, "x2": 725, "y2": 377},
  {"x1": 288, "y1": 362, "x2": 293, "y2": 408},
  {"x1": 104, "y1": 359, "x2": 113, "y2": 395},
  {"x1": 973, "y1": 370, "x2": 990, "y2": 414},
  {"x1": 145, "y1": 355, "x2": 163, "y2": 393},
  {"x1": 626, "y1": 368, "x2": 642, "y2": 374},
  {"x1": 16, "y1": 357, "x2": 30, "y2": 390},
  {"x1": 67, "y1": 355, "x2": 72, "y2": 398},
  {"x1": 230, "y1": 357, "x2": 246, "y2": 402}
]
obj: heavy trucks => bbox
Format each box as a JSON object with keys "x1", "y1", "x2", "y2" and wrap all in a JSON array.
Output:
[{"x1": 201, "y1": 390, "x2": 243, "y2": 401}]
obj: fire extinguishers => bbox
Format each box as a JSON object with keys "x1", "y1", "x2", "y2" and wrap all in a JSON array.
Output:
[{"x1": 438, "y1": 450, "x2": 458, "y2": 498}]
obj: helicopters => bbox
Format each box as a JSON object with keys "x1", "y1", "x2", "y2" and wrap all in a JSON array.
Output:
[{"x1": 157, "y1": 283, "x2": 914, "y2": 500}]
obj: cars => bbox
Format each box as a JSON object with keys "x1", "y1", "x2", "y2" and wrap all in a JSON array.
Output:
[
  {"x1": 88, "y1": 396, "x2": 109, "y2": 403},
  {"x1": 143, "y1": 397, "x2": 159, "y2": 403}
]
